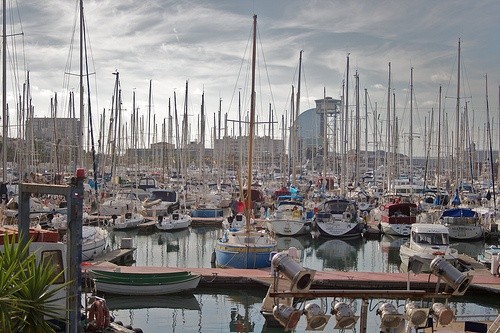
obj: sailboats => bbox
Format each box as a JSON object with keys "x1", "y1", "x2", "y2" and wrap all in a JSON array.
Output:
[{"x1": 0, "y1": 0, "x2": 500, "y2": 333}]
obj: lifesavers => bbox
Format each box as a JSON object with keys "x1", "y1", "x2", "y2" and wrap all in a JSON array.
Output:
[
  {"x1": 379, "y1": 205, "x2": 386, "y2": 211},
  {"x1": 52, "y1": 195, "x2": 58, "y2": 200},
  {"x1": 58, "y1": 195, "x2": 63, "y2": 200},
  {"x1": 291, "y1": 210, "x2": 300, "y2": 219},
  {"x1": 345, "y1": 212, "x2": 352, "y2": 221},
  {"x1": 433, "y1": 251, "x2": 445, "y2": 256}
]
